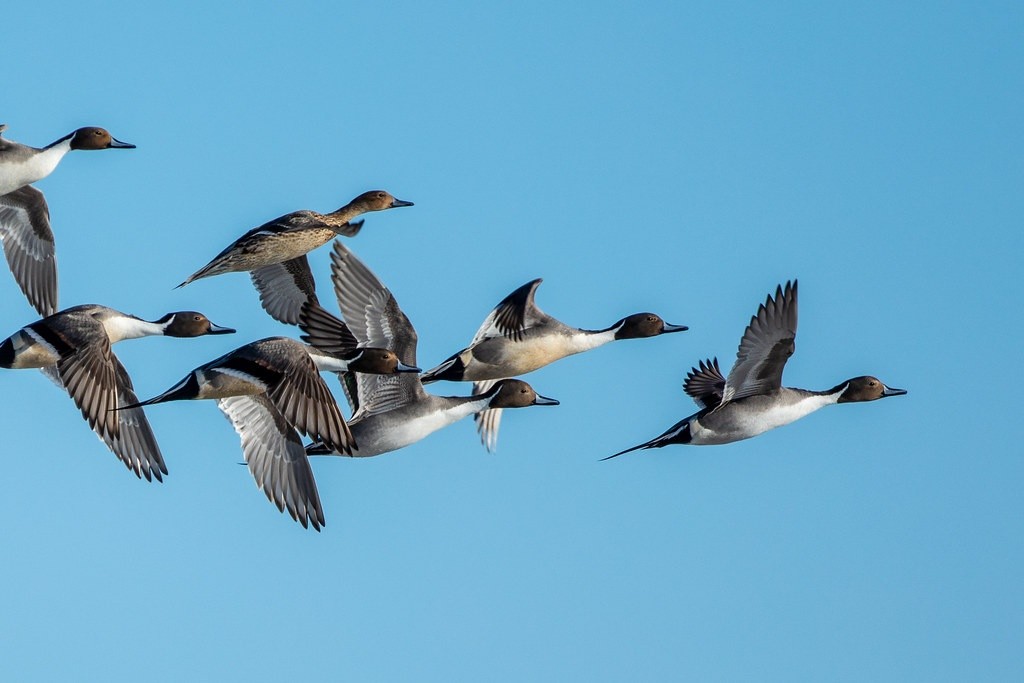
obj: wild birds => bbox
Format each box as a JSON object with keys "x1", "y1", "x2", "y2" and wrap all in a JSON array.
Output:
[
  {"x1": 0, "y1": 293, "x2": 235, "y2": 483},
  {"x1": 107, "y1": 190, "x2": 425, "y2": 532},
  {"x1": 237, "y1": 239, "x2": 688, "y2": 459},
  {"x1": 0, "y1": 127, "x2": 138, "y2": 315},
  {"x1": 598, "y1": 279, "x2": 906, "y2": 463}
]
